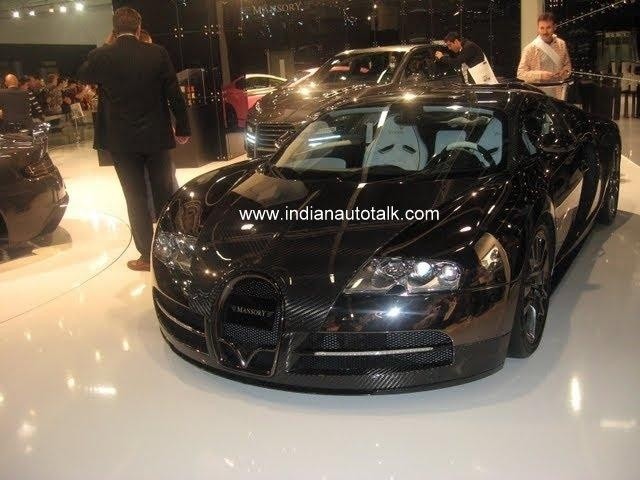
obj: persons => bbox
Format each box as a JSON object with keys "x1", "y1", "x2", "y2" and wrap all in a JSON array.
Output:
[
  {"x1": 78, "y1": 6, "x2": 192, "y2": 273},
  {"x1": 133, "y1": 28, "x2": 181, "y2": 226},
  {"x1": 0, "y1": 72, "x2": 99, "y2": 136},
  {"x1": 516, "y1": 12, "x2": 575, "y2": 102},
  {"x1": 434, "y1": 30, "x2": 490, "y2": 84}
]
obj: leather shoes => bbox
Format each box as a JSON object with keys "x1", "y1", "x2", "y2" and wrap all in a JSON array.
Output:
[{"x1": 127, "y1": 259, "x2": 150, "y2": 271}]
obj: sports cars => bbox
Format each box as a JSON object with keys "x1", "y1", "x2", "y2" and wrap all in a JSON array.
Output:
[{"x1": 149, "y1": 86, "x2": 623, "y2": 396}]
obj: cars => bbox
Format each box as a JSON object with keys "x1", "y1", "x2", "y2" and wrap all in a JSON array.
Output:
[
  {"x1": 221, "y1": 72, "x2": 288, "y2": 133},
  {"x1": 0, "y1": 127, "x2": 72, "y2": 245}
]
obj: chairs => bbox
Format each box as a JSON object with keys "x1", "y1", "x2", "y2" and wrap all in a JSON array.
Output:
[
  {"x1": 360, "y1": 111, "x2": 539, "y2": 168},
  {"x1": 376, "y1": 54, "x2": 456, "y2": 82}
]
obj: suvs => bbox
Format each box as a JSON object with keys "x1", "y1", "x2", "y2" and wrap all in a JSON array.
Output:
[{"x1": 242, "y1": 41, "x2": 466, "y2": 162}]
